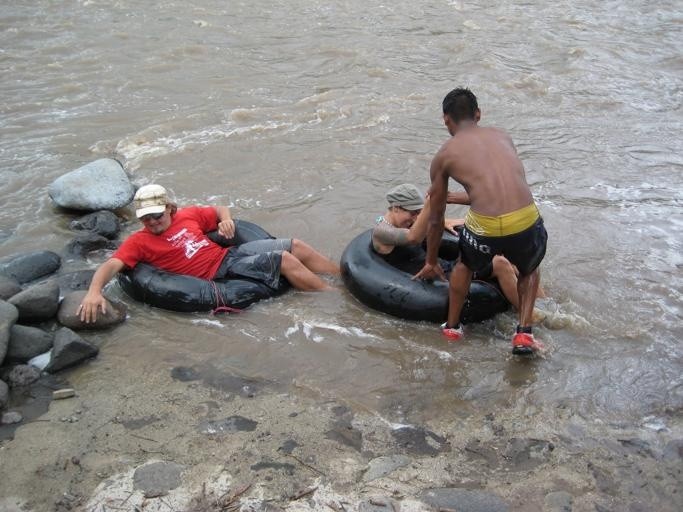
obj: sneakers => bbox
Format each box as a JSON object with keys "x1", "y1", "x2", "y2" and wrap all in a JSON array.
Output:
[
  {"x1": 511, "y1": 332, "x2": 545, "y2": 355},
  {"x1": 440, "y1": 322, "x2": 464, "y2": 340}
]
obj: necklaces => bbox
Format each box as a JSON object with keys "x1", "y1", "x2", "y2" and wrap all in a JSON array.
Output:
[{"x1": 381, "y1": 217, "x2": 395, "y2": 228}]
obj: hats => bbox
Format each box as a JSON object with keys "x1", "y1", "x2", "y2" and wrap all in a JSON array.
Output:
[
  {"x1": 386, "y1": 182, "x2": 426, "y2": 211},
  {"x1": 133, "y1": 183, "x2": 172, "y2": 219}
]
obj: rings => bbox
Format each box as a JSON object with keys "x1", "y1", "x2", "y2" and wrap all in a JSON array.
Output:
[{"x1": 79, "y1": 304, "x2": 82, "y2": 307}]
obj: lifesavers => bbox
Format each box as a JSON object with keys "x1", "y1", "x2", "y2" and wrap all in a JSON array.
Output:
[
  {"x1": 340, "y1": 225, "x2": 511, "y2": 323},
  {"x1": 118, "y1": 218, "x2": 288, "y2": 312}
]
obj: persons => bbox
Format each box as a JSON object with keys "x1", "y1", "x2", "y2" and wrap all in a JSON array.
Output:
[
  {"x1": 409, "y1": 86, "x2": 549, "y2": 358},
  {"x1": 368, "y1": 182, "x2": 575, "y2": 331},
  {"x1": 74, "y1": 182, "x2": 345, "y2": 324}
]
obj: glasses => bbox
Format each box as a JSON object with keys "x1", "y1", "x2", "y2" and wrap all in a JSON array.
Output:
[{"x1": 142, "y1": 213, "x2": 165, "y2": 220}]
